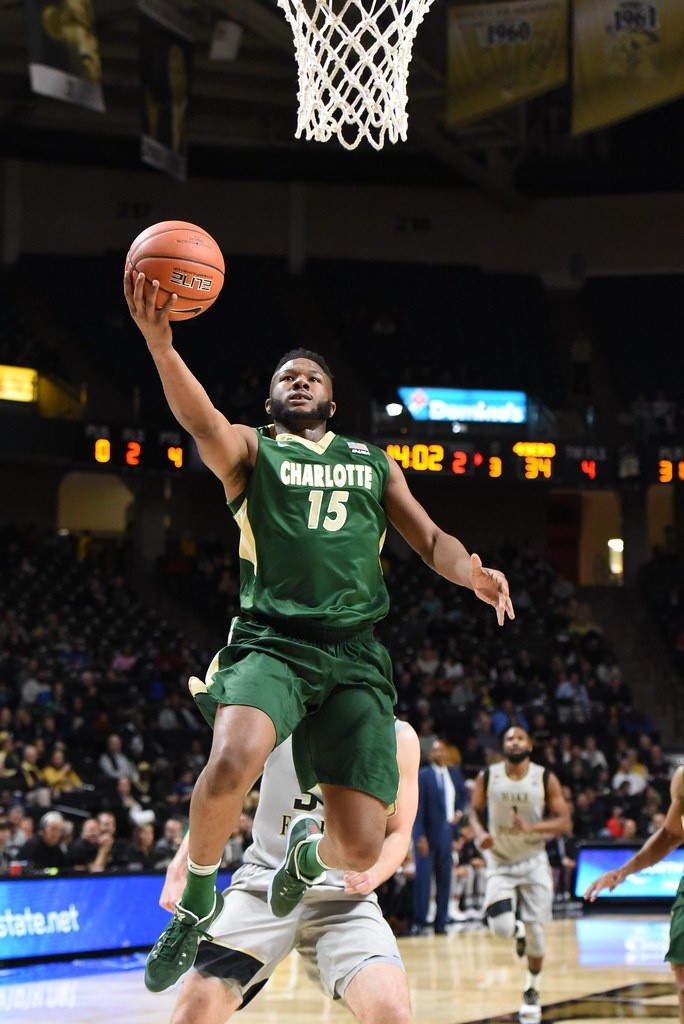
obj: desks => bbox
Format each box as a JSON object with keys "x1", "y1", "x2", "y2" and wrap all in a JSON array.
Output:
[
  {"x1": 571, "y1": 839, "x2": 684, "y2": 914},
  {"x1": 0, "y1": 867, "x2": 239, "y2": 969}
]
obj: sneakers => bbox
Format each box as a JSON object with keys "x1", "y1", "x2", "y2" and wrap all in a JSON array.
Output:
[
  {"x1": 267, "y1": 815, "x2": 324, "y2": 919},
  {"x1": 144, "y1": 885, "x2": 224, "y2": 993}
]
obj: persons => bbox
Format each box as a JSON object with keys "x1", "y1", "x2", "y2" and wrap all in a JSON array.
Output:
[
  {"x1": 411, "y1": 738, "x2": 472, "y2": 936},
  {"x1": 584, "y1": 764, "x2": 684, "y2": 1024},
  {"x1": 122, "y1": 271, "x2": 517, "y2": 995},
  {"x1": 470, "y1": 727, "x2": 571, "y2": 1024},
  {"x1": 160, "y1": 715, "x2": 420, "y2": 1024},
  {"x1": 0, "y1": 532, "x2": 684, "y2": 875}
]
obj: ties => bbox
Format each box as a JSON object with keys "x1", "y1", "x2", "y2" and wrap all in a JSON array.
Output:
[{"x1": 438, "y1": 773, "x2": 445, "y2": 805}]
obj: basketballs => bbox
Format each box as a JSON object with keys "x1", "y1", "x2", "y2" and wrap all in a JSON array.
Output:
[{"x1": 124, "y1": 217, "x2": 227, "y2": 324}]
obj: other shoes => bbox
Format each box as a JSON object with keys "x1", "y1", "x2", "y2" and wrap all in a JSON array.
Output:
[
  {"x1": 514, "y1": 920, "x2": 526, "y2": 958},
  {"x1": 518, "y1": 989, "x2": 540, "y2": 1014}
]
